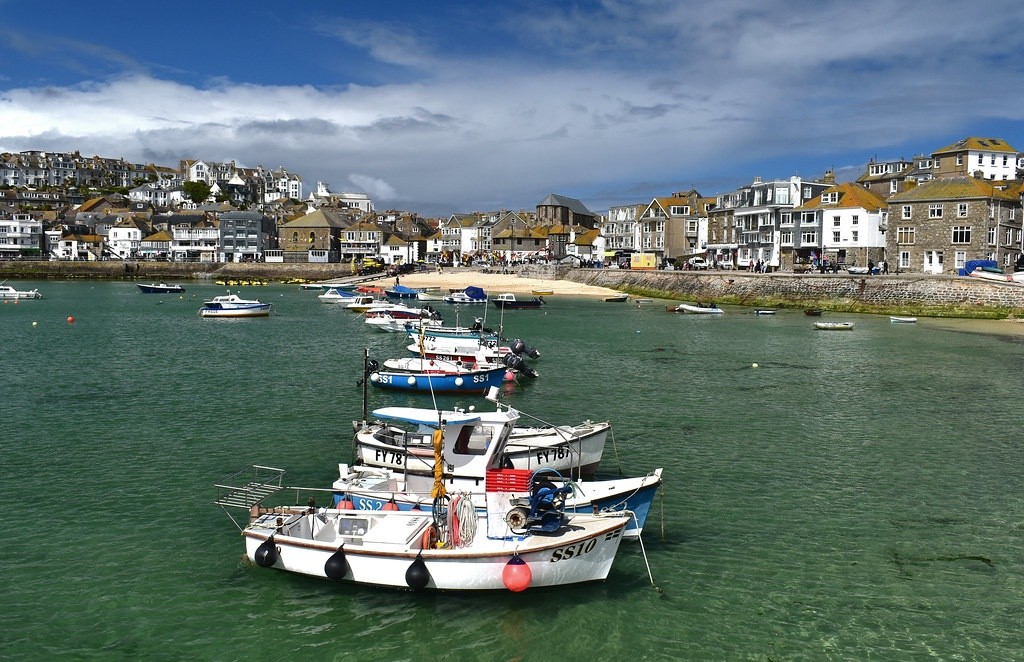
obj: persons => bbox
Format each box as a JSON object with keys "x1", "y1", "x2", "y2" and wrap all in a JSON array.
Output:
[
  {"x1": 396, "y1": 275, "x2": 399, "y2": 286},
  {"x1": 426, "y1": 265, "x2": 443, "y2": 274},
  {"x1": 820, "y1": 260, "x2": 839, "y2": 273},
  {"x1": 580, "y1": 259, "x2": 631, "y2": 269},
  {"x1": 868, "y1": 260, "x2": 874, "y2": 275},
  {"x1": 750, "y1": 259, "x2": 769, "y2": 273},
  {"x1": 713, "y1": 258, "x2": 718, "y2": 270},
  {"x1": 883, "y1": 260, "x2": 890, "y2": 275}
]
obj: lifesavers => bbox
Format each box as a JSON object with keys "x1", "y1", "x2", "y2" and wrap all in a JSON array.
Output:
[{"x1": 423, "y1": 526, "x2": 437, "y2": 549}]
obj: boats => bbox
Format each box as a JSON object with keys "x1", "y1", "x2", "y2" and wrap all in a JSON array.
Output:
[
  {"x1": 889, "y1": 315, "x2": 918, "y2": 323},
  {"x1": 803, "y1": 309, "x2": 823, "y2": 315},
  {"x1": 201, "y1": 302, "x2": 273, "y2": 317},
  {"x1": 215, "y1": 277, "x2": 268, "y2": 286},
  {"x1": 848, "y1": 267, "x2": 881, "y2": 274},
  {"x1": 0, "y1": 284, "x2": 42, "y2": 300},
  {"x1": 299, "y1": 282, "x2": 555, "y2": 394},
  {"x1": 679, "y1": 303, "x2": 724, "y2": 314},
  {"x1": 753, "y1": 307, "x2": 778, "y2": 314},
  {"x1": 601, "y1": 294, "x2": 628, "y2": 302},
  {"x1": 243, "y1": 505, "x2": 632, "y2": 590},
  {"x1": 203, "y1": 295, "x2": 260, "y2": 308},
  {"x1": 137, "y1": 282, "x2": 185, "y2": 293},
  {"x1": 814, "y1": 321, "x2": 857, "y2": 330},
  {"x1": 351, "y1": 414, "x2": 610, "y2": 480},
  {"x1": 755, "y1": 265, "x2": 780, "y2": 272},
  {"x1": 279, "y1": 278, "x2": 315, "y2": 284},
  {"x1": 332, "y1": 404, "x2": 666, "y2": 541}
]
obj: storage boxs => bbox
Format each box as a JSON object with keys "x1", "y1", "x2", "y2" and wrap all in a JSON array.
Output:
[{"x1": 486, "y1": 468, "x2": 533, "y2": 540}]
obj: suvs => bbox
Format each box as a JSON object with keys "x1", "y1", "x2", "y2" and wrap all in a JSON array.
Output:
[
  {"x1": 660, "y1": 257, "x2": 683, "y2": 270},
  {"x1": 689, "y1": 256, "x2": 709, "y2": 270}
]
